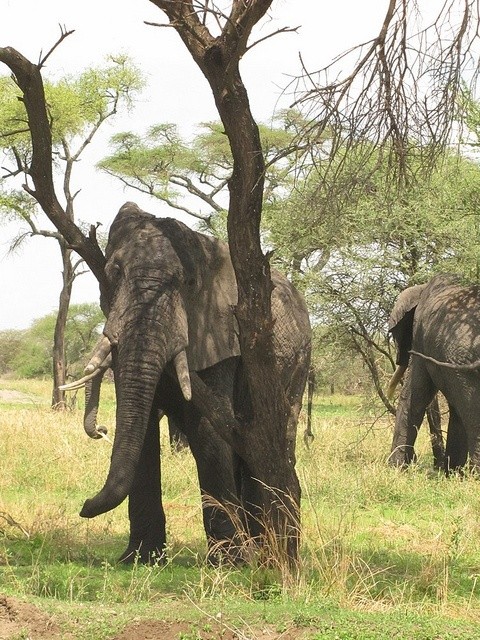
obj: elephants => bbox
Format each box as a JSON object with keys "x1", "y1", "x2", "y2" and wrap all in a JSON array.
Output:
[
  {"x1": 57, "y1": 334, "x2": 191, "y2": 456},
  {"x1": 387, "y1": 273, "x2": 480, "y2": 480},
  {"x1": 79, "y1": 200, "x2": 315, "y2": 569}
]
